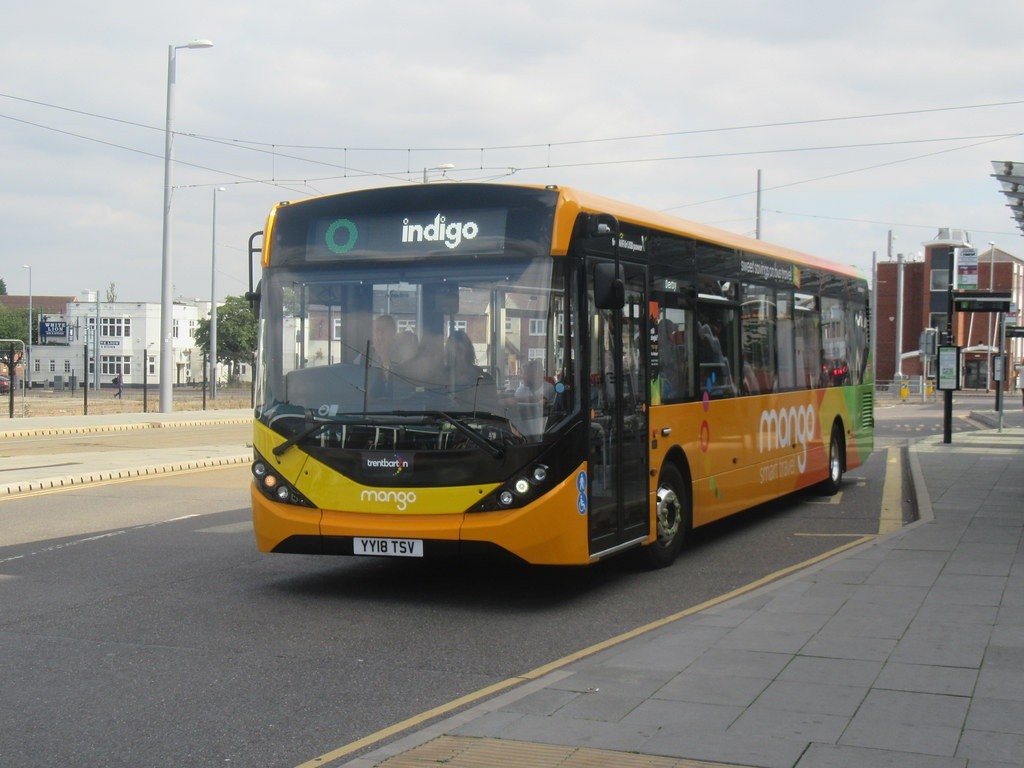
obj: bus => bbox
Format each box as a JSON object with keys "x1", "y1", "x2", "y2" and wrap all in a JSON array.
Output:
[{"x1": 252, "y1": 182, "x2": 877, "y2": 565}]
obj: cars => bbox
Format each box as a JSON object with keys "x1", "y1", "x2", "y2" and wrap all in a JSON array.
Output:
[{"x1": 0, "y1": 375, "x2": 15, "y2": 394}]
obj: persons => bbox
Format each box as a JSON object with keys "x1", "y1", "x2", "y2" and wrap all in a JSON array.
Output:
[
  {"x1": 113, "y1": 372, "x2": 124, "y2": 399},
  {"x1": 350, "y1": 311, "x2": 846, "y2": 411}
]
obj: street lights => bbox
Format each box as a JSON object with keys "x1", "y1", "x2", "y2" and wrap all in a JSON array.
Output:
[
  {"x1": 210, "y1": 187, "x2": 227, "y2": 401},
  {"x1": 159, "y1": 41, "x2": 213, "y2": 412},
  {"x1": 986, "y1": 240, "x2": 995, "y2": 393},
  {"x1": 23, "y1": 264, "x2": 33, "y2": 389}
]
